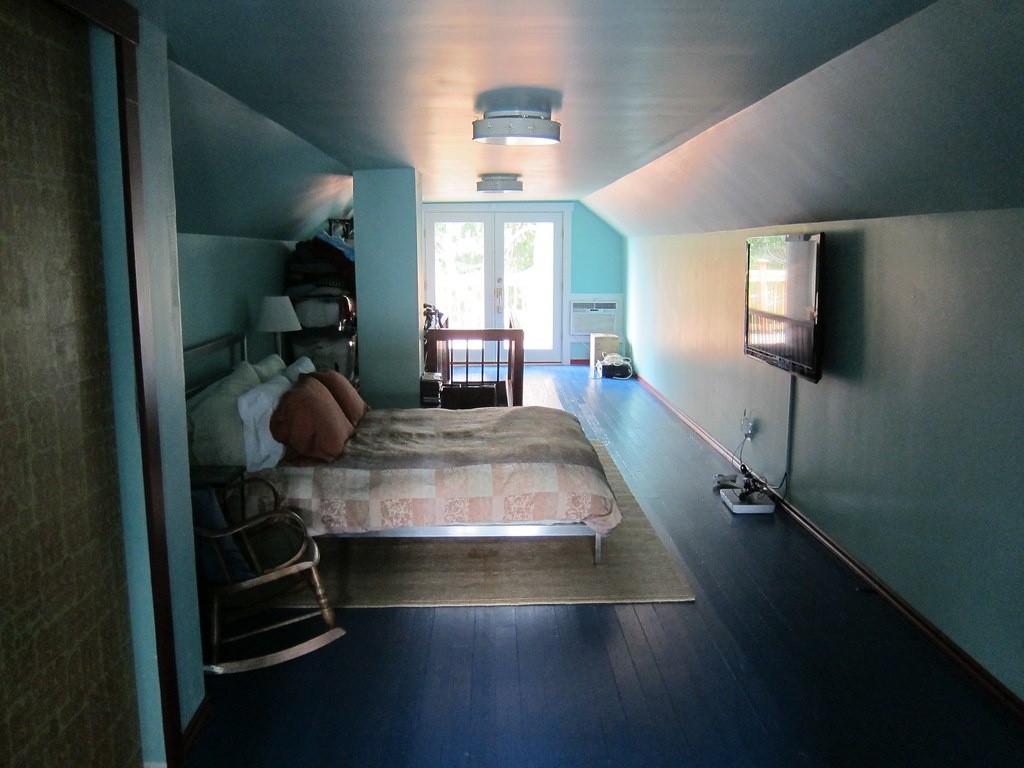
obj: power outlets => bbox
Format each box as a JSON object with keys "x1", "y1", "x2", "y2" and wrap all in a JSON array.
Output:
[{"x1": 738, "y1": 417, "x2": 755, "y2": 442}]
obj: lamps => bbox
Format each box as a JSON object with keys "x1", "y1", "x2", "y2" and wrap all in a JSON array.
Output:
[
  {"x1": 477, "y1": 174, "x2": 523, "y2": 193},
  {"x1": 472, "y1": 110, "x2": 561, "y2": 145},
  {"x1": 255, "y1": 296, "x2": 302, "y2": 359}
]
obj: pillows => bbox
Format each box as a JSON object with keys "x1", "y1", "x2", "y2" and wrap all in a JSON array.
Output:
[{"x1": 188, "y1": 353, "x2": 370, "y2": 472}]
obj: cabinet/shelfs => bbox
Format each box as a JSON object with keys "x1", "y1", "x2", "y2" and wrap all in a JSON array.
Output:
[{"x1": 424, "y1": 313, "x2": 453, "y2": 385}]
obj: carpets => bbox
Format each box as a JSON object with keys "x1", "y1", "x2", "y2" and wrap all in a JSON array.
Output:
[{"x1": 208, "y1": 441, "x2": 697, "y2": 608}]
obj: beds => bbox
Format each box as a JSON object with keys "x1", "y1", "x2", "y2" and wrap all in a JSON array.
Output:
[{"x1": 182, "y1": 331, "x2": 623, "y2": 566}]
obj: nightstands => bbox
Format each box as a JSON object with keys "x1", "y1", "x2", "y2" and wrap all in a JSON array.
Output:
[{"x1": 188, "y1": 462, "x2": 246, "y2": 527}]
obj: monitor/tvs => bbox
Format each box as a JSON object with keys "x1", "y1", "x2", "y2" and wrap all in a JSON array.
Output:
[{"x1": 743, "y1": 232, "x2": 828, "y2": 383}]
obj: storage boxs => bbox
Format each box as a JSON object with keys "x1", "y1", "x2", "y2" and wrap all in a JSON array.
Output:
[
  {"x1": 596, "y1": 357, "x2": 629, "y2": 378},
  {"x1": 589, "y1": 332, "x2": 620, "y2": 369},
  {"x1": 421, "y1": 370, "x2": 444, "y2": 409}
]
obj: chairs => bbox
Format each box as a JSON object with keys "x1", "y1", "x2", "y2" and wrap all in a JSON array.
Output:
[{"x1": 191, "y1": 476, "x2": 348, "y2": 675}]
